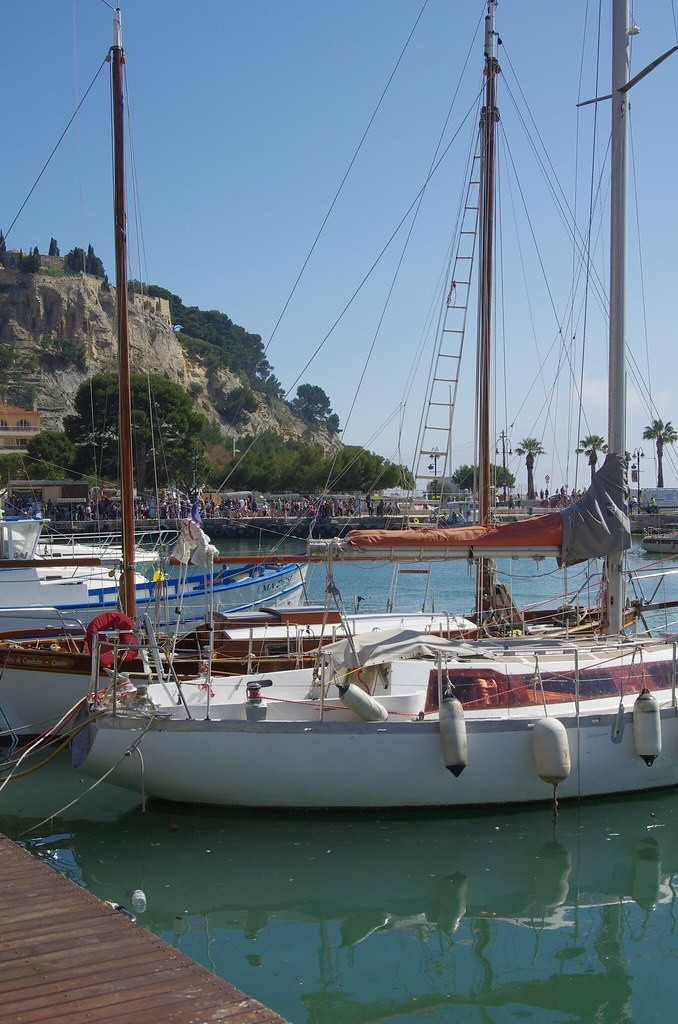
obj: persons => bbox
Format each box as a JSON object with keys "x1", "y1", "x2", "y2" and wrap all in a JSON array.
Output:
[
  {"x1": 9, "y1": 487, "x2": 397, "y2": 520},
  {"x1": 422, "y1": 484, "x2": 657, "y2": 522}
]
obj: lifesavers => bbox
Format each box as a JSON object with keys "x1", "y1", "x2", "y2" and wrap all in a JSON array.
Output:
[{"x1": 87, "y1": 612, "x2": 139, "y2": 666}]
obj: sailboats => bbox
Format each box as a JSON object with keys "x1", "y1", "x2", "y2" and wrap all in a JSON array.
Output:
[{"x1": 0, "y1": 1, "x2": 678, "y2": 823}]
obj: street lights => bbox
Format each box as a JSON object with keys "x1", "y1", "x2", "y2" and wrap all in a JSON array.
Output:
[
  {"x1": 632, "y1": 446, "x2": 645, "y2": 514},
  {"x1": 430, "y1": 446, "x2": 441, "y2": 507},
  {"x1": 191, "y1": 445, "x2": 201, "y2": 495},
  {"x1": 495, "y1": 430, "x2": 514, "y2": 508}
]
